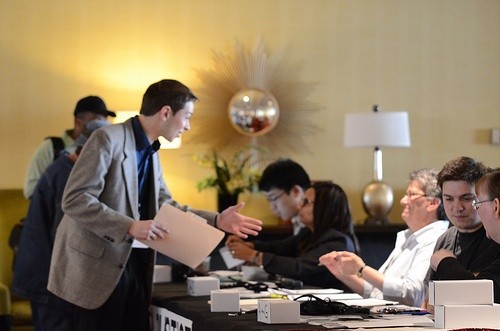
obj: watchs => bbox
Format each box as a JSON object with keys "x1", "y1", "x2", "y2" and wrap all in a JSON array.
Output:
[
  {"x1": 356, "y1": 264, "x2": 367, "y2": 276},
  {"x1": 253, "y1": 251, "x2": 260, "y2": 266}
]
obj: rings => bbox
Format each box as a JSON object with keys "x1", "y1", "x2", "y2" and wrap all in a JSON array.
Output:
[{"x1": 231, "y1": 250, "x2": 235, "y2": 255}]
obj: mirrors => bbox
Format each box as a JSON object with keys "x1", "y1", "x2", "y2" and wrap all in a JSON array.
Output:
[{"x1": 186, "y1": 39, "x2": 328, "y2": 176}]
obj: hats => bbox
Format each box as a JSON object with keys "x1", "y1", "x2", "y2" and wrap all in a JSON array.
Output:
[
  {"x1": 74, "y1": 96, "x2": 117, "y2": 118},
  {"x1": 75, "y1": 120, "x2": 114, "y2": 147}
]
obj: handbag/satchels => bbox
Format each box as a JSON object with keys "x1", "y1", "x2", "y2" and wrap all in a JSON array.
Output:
[{"x1": 7, "y1": 136, "x2": 65, "y2": 249}]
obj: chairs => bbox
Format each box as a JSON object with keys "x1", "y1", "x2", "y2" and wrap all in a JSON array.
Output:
[{"x1": 248, "y1": 223, "x2": 409, "y2": 271}]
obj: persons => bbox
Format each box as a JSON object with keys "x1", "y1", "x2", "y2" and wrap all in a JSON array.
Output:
[
  {"x1": 14, "y1": 119, "x2": 115, "y2": 331},
  {"x1": 23, "y1": 96, "x2": 117, "y2": 199},
  {"x1": 319, "y1": 169, "x2": 449, "y2": 307},
  {"x1": 423, "y1": 156, "x2": 500, "y2": 303},
  {"x1": 259, "y1": 156, "x2": 311, "y2": 237},
  {"x1": 226, "y1": 180, "x2": 360, "y2": 289},
  {"x1": 47, "y1": 79, "x2": 262, "y2": 331}
]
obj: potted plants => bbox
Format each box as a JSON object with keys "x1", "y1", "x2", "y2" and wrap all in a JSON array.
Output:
[{"x1": 193, "y1": 144, "x2": 267, "y2": 214}]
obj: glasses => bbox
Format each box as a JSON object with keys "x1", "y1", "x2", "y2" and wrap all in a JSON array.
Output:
[
  {"x1": 471, "y1": 198, "x2": 493, "y2": 211},
  {"x1": 267, "y1": 190, "x2": 288, "y2": 202},
  {"x1": 300, "y1": 198, "x2": 316, "y2": 208},
  {"x1": 405, "y1": 190, "x2": 424, "y2": 199}
]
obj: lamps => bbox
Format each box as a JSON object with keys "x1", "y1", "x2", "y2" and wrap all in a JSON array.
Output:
[{"x1": 342, "y1": 104, "x2": 411, "y2": 224}]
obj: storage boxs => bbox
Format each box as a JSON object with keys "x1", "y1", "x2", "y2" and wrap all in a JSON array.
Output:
[
  {"x1": 428, "y1": 279, "x2": 500, "y2": 329},
  {"x1": 210, "y1": 290, "x2": 240, "y2": 313},
  {"x1": 187, "y1": 276, "x2": 220, "y2": 297},
  {"x1": 257, "y1": 299, "x2": 301, "y2": 324}
]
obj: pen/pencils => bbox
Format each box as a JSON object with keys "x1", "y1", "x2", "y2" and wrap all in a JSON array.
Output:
[
  {"x1": 378, "y1": 307, "x2": 431, "y2": 315},
  {"x1": 318, "y1": 261, "x2": 322, "y2": 266}
]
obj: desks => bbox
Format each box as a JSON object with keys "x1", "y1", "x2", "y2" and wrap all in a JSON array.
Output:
[{"x1": 148, "y1": 279, "x2": 436, "y2": 331}]
